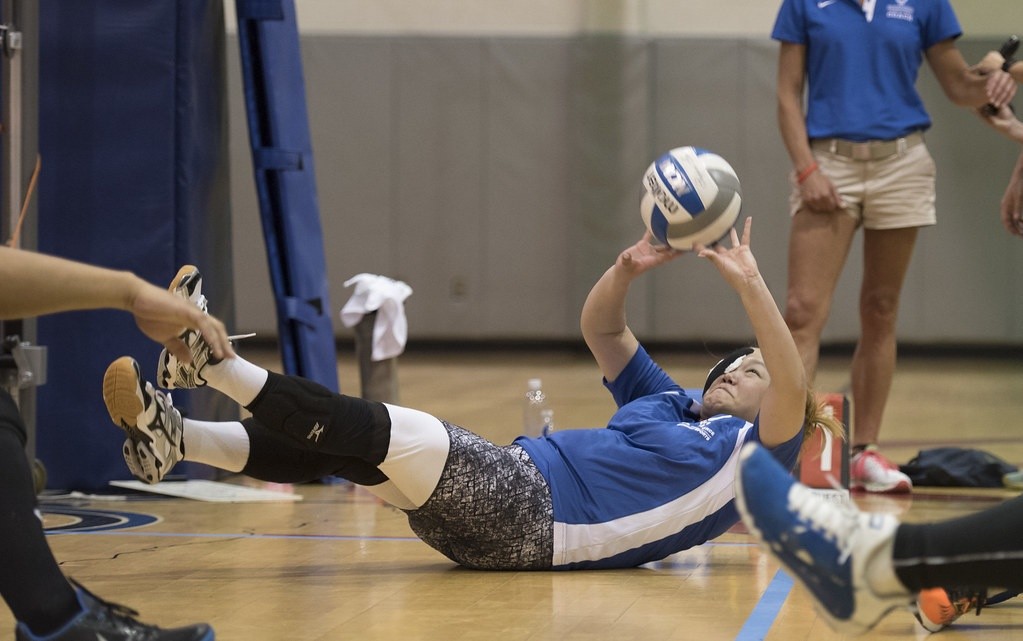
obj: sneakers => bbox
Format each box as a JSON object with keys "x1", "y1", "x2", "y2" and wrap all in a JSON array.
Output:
[
  {"x1": 102, "y1": 356, "x2": 182, "y2": 483},
  {"x1": 913, "y1": 587, "x2": 986, "y2": 635},
  {"x1": 157, "y1": 265, "x2": 257, "y2": 391},
  {"x1": 849, "y1": 445, "x2": 912, "y2": 495},
  {"x1": 733, "y1": 440, "x2": 913, "y2": 635},
  {"x1": 16, "y1": 578, "x2": 215, "y2": 641}
]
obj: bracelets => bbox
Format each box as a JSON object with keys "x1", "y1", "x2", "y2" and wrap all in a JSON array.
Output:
[{"x1": 797, "y1": 163, "x2": 816, "y2": 180}]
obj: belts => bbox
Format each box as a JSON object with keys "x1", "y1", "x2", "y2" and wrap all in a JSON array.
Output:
[{"x1": 811, "y1": 130, "x2": 923, "y2": 160}]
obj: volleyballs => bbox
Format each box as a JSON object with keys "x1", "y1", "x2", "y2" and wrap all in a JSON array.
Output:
[{"x1": 638, "y1": 145, "x2": 744, "y2": 255}]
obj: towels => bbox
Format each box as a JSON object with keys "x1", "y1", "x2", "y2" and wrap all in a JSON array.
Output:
[{"x1": 340, "y1": 271, "x2": 413, "y2": 361}]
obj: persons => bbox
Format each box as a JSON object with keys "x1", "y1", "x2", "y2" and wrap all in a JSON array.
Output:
[
  {"x1": 103, "y1": 215, "x2": 846, "y2": 571},
  {"x1": 0, "y1": 247, "x2": 236, "y2": 641},
  {"x1": 771, "y1": 0, "x2": 1017, "y2": 495},
  {"x1": 974, "y1": 51, "x2": 1023, "y2": 237},
  {"x1": 733, "y1": 441, "x2": 1023, "y2": 633}
]
